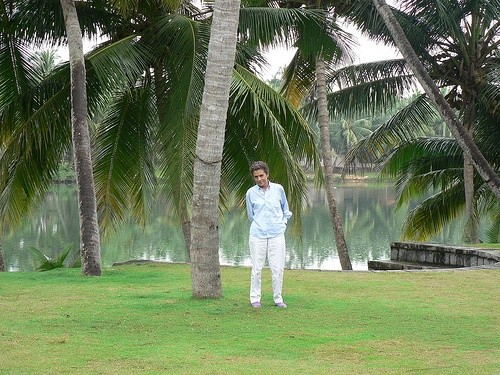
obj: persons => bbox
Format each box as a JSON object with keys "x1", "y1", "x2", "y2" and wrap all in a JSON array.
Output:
[{"x1": 244, "y1": 161, "x2": 294, "y2": 308}]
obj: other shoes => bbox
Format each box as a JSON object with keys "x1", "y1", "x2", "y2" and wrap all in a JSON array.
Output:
[
  {"x1": 253, "y1": 302, "x2": 260, "y2": 308},
  {"x1": 275, "y1": 302, "x2": 287, "y2": 307}
]
